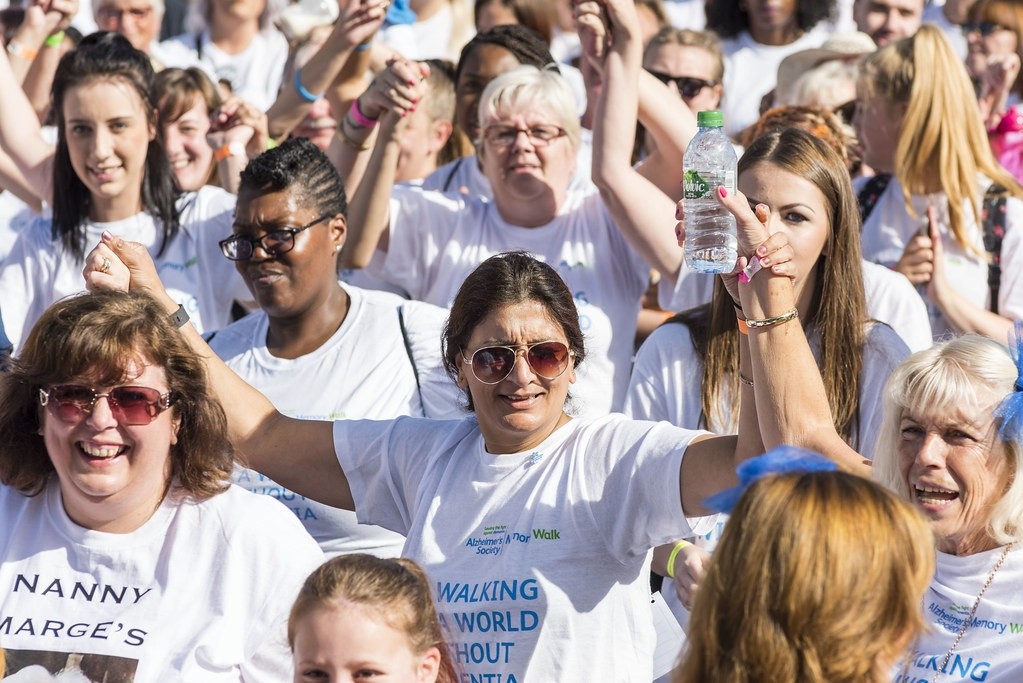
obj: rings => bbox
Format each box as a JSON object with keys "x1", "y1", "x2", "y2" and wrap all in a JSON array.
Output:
[{"x1": 101, "y1": 258, "x2": 111, "y2": 272}]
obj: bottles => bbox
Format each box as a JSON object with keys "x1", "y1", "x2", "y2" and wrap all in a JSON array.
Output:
[{"x1": 682, "y1": 110, "x2": 739, "y2": 275}]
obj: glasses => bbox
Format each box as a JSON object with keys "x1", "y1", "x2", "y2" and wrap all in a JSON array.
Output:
[
  {"x1": 482, "y1": 122, "x2": 568, "y2": 147},
  {"x1": 649, "y1": 68, "x2": 718, "y2": 98},
  {"x1": 37, "y1": 382, "x2": 178, "y2": 427},
  {"x1": 456, "y1": 339, "x2": 574, "y2": 383},
  {"x1": 219, "y1": 211, "x2": 335, "y2": 261}
]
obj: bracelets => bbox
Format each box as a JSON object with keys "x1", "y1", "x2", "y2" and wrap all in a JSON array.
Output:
[
  {"x1": 746, "y1": 310, "x2": 798, "y2": 328},
  {"x1": 731, "y1": 299, "x2": 742, "y2": 311},
  {"x1": 336, "y1": 121, "x2": 370, "y2": 152},
  {"x1": 352, "y1": 99, "x2": 378, "y2": 128},
  {"x1": 166, "y1": 304, "x2": 190, "y2": 329},
  {"x1": 740, "y1": 376, "x2": 753, "y2": 387},
  {"x1": 44, "y1": 32, "x2": 66, "y2": 47},
  {"x1": 268, "y1": 137, "x2": 278, "y2": 150},
  {"x1": 737, "y1": 318, "x2": 748, "y2": 336},
  {"x1": 4, "y1": 37, "x2": 36, "y2": 61},
  {"x1": 667, "y1": 541, "x2": 691, "y2": 578},
  {"x1": 293, "y1": 71, "x2": 321, "y2": 102},
  {"x1": 213, "y1": 142, "x2": 246, "y2": 162}
]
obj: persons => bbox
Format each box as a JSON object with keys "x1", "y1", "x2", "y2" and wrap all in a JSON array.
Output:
[{"x1": 0, "y1": 0, "x2": 1023, "y2": 683}]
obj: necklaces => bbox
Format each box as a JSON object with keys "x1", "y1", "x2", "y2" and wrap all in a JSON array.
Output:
[{"x1": 904, "y1": 543, "x2": 1012, "y2": 683}]
